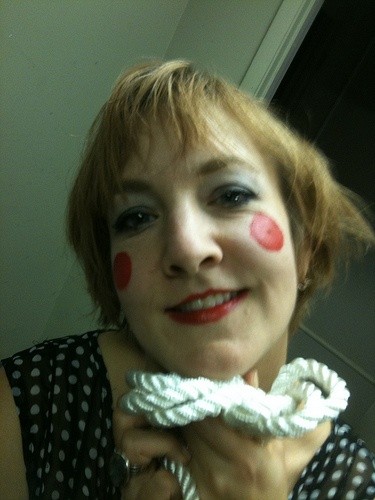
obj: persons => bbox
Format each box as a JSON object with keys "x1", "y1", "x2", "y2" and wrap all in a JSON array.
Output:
[{"x1": 0, "y1": 58, "x2": 375, "y2": 500}]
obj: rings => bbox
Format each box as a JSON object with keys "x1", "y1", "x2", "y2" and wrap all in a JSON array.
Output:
[{"x1": 107, "y1": 451, "x2": 141, "y2": 487}]
